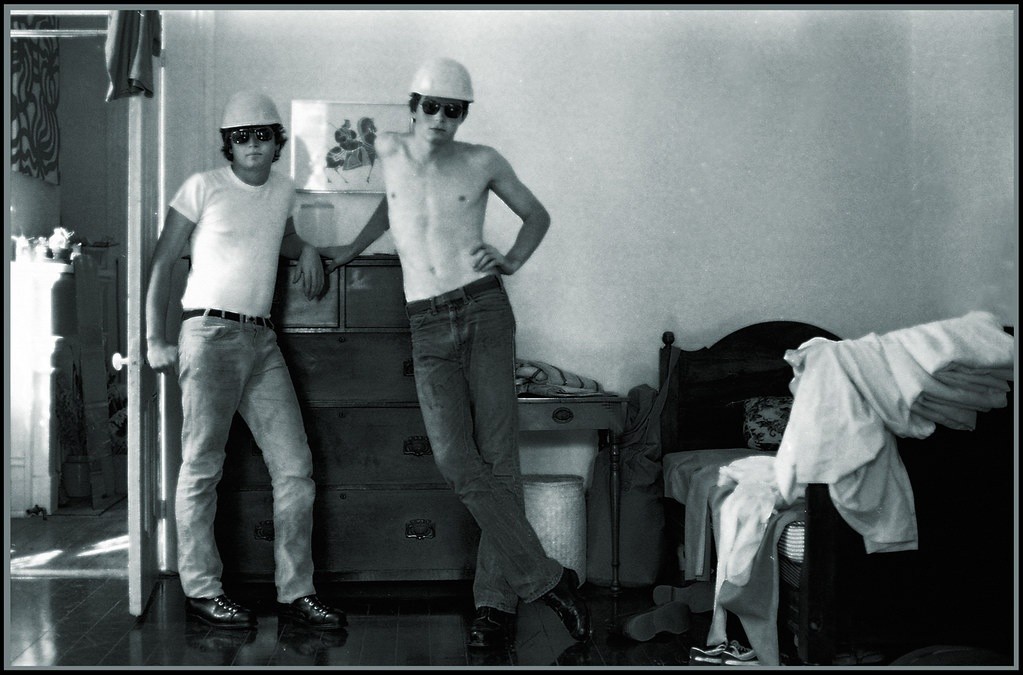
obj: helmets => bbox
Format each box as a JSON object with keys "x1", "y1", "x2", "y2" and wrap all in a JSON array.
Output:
[
  {"x1": 221, "y1": 91, "x2": 282, "y2": 129},
  {"x1": 412, "y1": 58, "x2": 474, "y2": 103}
]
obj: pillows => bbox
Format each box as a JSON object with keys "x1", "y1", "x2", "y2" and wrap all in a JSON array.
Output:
[{"x1": 730, "y1": 397, "x2": 792, "y2": 451}]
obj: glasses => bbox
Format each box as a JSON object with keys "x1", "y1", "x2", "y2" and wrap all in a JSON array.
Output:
[
  {"x1": 230, "y1": 127, "x2": 275, "y2": 143},
  {"x1": 417, "y1": 100, "x2": 463, "y2": 119}
]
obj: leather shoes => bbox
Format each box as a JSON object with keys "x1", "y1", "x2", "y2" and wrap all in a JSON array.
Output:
[
  {"x1": 468, "y1": 607, "x2": 507, "y2": 646},
  {"x1": 184, "y1": 594, "x2": 258, "y2": 627},
  {"x1": 542, "y1": 568, "x2": 594, "y2": 643},
  {"x1": 276, "y1": 596, "x2": 348, "y2": 630}
]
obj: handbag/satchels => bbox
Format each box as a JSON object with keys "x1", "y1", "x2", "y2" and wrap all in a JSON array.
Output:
[{"x1": 623, "y1": 346, "x2": 681, "y2": 459}]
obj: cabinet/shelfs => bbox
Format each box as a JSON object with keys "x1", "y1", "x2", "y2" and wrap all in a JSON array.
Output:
[{"x1": 179, "y1": 254, "x2": 631, "y2": 592}]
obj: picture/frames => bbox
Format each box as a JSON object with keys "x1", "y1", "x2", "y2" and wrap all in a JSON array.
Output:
[{"x1": 290, "y1": 100, "x2": 413, "y2": 193}]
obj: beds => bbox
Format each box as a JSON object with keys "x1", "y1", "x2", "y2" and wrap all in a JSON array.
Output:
[{"x1": 656, "y1": 321, "x2": 1017, "y2": 670}]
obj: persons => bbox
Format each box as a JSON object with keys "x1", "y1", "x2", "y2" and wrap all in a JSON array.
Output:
[
  {"x1": 313, "y1": 58, "x2": 592, "y2": 657},
  {"x1": 144, "y1": 87, "x2": 345, "y2": 632}
]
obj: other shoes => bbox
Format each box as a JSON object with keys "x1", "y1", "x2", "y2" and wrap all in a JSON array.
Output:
[
  {"x1": 604, "y1": 601, "x2": 693, "y2": 640},
  {"x1": 831, "y1": 652, "x2": 857, "y2": 664},
  {"x1": 653, "y1": 582, "x2": 715, "y2": 612},
  {"x1": 856, "y1": 648, "x2": 884, "y2": 663},
  {"x1": 689, "y1": 640, "x2": 760, "y2": 665}
]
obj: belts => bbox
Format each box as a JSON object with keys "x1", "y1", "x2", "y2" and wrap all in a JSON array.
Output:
[
  {"x1": 407, "y1": 280, "x2": 499, "y2": 315},
  {"x1": 183, "y1": 309, "x2": 274, "y2": 329}
]
